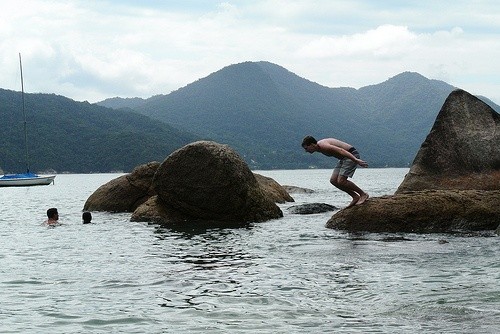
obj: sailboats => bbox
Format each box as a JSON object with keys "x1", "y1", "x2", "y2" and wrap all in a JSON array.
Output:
[{"x1": 0, "y1": 52, "x2": 57, "y2": 187}]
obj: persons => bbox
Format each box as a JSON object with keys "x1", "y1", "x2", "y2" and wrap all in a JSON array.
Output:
[
  {"x1": 40, "y1": 208, "x2": 60, "y2": 226},
  {"x1": 301, "y1": 135, "x2": 369, "y2": 207},
  {"x1": 82, "y1": 212, "x2": 93, "y2": 224}
]
